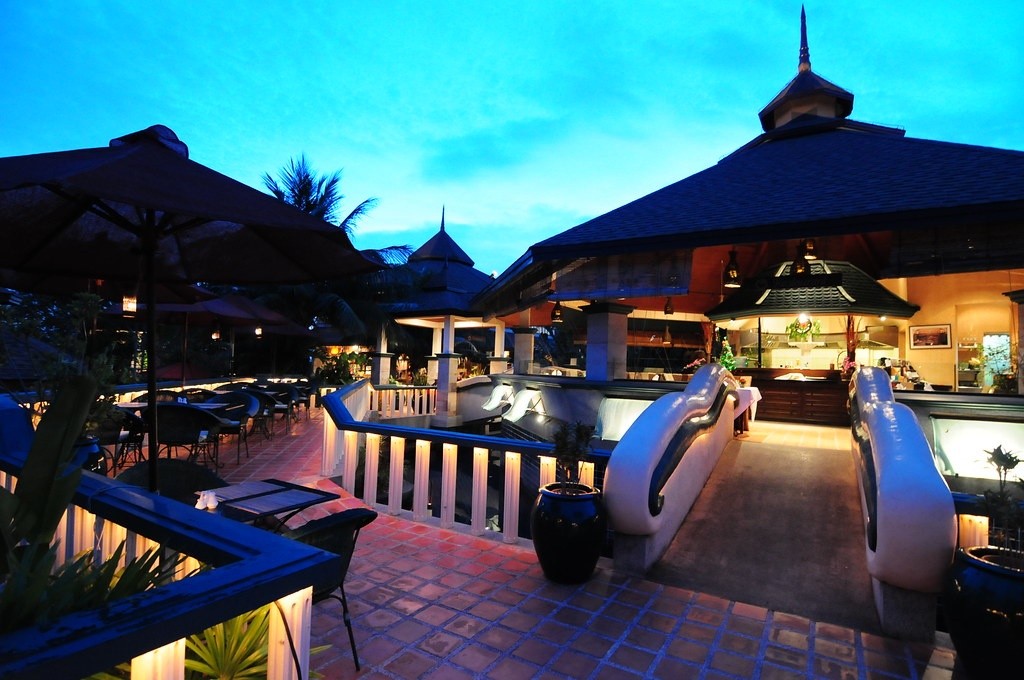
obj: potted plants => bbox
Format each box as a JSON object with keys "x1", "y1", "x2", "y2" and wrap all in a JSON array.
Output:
[
  {"x1": 530, "y1": 416, "x2": 609, "y2": 584},
  {"x1": 947, "y1": 446, "x2": 1024, "y2": 620}
]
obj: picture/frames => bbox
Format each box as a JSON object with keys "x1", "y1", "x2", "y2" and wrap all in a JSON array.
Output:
[{"x1": 909, "y1": 324, "x2": 953, "y2": 349}]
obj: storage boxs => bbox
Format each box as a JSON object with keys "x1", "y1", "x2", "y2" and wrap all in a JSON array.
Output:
[{"x1": 866, "y1": 326, "x2": 899, "y2": 347}]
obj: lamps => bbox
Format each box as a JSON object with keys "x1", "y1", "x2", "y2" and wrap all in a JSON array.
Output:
[
  {"x1": 790, "y1": 241, "x2": 811, "y2": 276},
  {"x1": 661, "y1": 326, "x2": 671, "y2": 344},
  {"x1": 550, "y1": 302, "x2": 563, "y2": 324},
  {"x1": 722, "y1": 246, "x2": 741, "y2": 287},
  {"x1": 663, "y1": 298, "x2": 674, "y2": 315}
]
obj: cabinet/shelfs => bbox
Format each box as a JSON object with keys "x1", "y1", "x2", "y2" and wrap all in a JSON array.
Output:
[{"x1": 955, "y1": 342, "x2": 984, "y2": 392}]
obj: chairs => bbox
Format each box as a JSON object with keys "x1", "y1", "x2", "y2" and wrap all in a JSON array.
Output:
[
  {"x1": 98, "y1": 377, "x2": 313, "y2": 472},
  {"x1": 281, "y1": 507, "x2": 378, "y2": 672},
  {"x1": 112, "y1": 459, "x2": 230, "y2": 507}
]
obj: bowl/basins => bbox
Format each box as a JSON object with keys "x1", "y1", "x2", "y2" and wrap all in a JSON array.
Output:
[{"x1": 930, "y1": 385, "x2": 952, "y2": 391}]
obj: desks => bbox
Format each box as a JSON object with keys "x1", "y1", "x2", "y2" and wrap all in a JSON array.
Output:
[
  {"x1": 193, "y1": 478, "x2": 341, "y2": 534},
  {"x1": 117, "y1": 401, "x2": 230, "y2": 411}
]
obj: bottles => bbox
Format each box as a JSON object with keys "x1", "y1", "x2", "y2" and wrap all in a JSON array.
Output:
[{"x1": 207, "y1": 492, "x2": 218, "y2": 510}]
